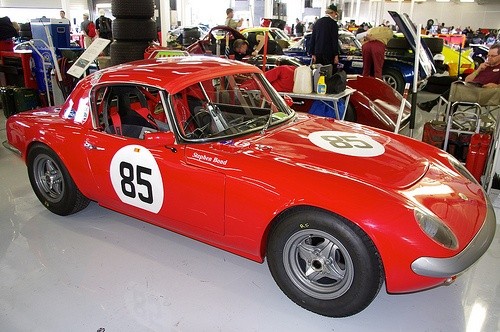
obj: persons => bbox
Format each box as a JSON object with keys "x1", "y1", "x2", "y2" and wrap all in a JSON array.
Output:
[
  {"x1": 246, "y1": 18, "x2": 250, "y2": 28},
  {"x1": 308, "y1": 3, "x2": 344, "y2": 74},
  {"x1": 70, "y1": 17, "x2": 80, "y2": 34},
  {"x1": 384, "y1": 21, "x2": 390, "y2": 27},
  {"x1": 424, "y1": 18, "x2": 500, "y2": 48},
  {"x1": 361, "y1": 24, "x2": 394, "y2": 78},
  {"x1": 294, "y1": 16, "x2": 318, "y2": 37},
  {"x1": 59, "y1": 10, "x2": 71, "y2": 32},
  {"x1": 80, "y1": 8, "x2": 113, "y2": 64},
  {"x1": 225, "y1": 7, "x2": 244, "y2": 32},
  {"x1": 355, "y1": 22, "x2": 373, "y2": 37},
  {"x1": 232, "y1": 35, "x2": 285, "y2": 61},
  {"x1": 416, "y1": 41, "x2": 500, "y2": 122},
  {"x1": 167, "y1": 23, "x2": 184, "y2": 42}
]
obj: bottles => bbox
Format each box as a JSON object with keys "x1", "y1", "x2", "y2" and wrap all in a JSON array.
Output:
[{"x1": 317, "y1": 75, "x2": 327, "y2": 95}]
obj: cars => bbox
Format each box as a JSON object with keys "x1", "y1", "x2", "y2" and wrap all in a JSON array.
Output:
[
  {"x1": 1, "y1": 49, "x2": 497, "y2": 318},
  {"x1": 0, "y1": 27, "x2": 490, "y2": 135}
]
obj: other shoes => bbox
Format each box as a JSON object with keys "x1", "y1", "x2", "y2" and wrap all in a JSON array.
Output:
[{"x1": 417, "y1": 102, "x2": 432, "y2": 112}]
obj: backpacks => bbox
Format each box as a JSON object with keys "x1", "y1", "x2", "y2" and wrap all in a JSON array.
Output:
[
  {"x1": 85, "y1": 21, "x2": 96, "y2": 37},
  {"x1": 98, "y1": 17, "x2": 110, "y2": 33}
]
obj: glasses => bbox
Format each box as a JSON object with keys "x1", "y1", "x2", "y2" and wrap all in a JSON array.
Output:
[{"x1": 486, "y1": 55, "x2": 499, "y2": 57}]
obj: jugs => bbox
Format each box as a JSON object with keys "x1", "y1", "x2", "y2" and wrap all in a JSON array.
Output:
[{"x1": 293, "y1": 63, "x2": 313, "y2": 94}]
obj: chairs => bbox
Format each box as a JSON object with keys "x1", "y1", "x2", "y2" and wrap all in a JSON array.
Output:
[{"x1": 103, "y1": 86, "x2": 155, "y2": 135}]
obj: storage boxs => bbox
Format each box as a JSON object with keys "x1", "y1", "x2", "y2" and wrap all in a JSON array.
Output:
[{"x1": 30, "y1": 17, "x2": 70, "y2": 47}]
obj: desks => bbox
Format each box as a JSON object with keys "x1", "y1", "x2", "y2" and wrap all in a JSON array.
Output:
[{"x1": 238, "y1": 86, "x2": 356, "y2": 121}]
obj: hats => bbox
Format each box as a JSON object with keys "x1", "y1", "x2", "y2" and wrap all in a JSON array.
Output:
[{"x1": 328, "y1": 4, "x2": 340, "y2": 14}]
